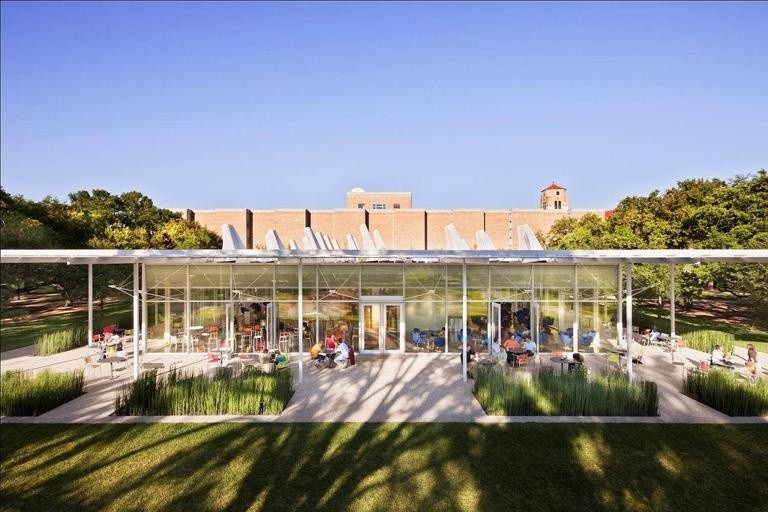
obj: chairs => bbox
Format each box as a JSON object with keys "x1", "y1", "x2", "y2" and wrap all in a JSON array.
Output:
[
  {"x1": 409, "y1": 317, "x2": 596, "y2": 376},
  {"x1": 603, "y1": 325, "x2": 762, "y2": 382},
  {"x1": 83, "y1": 312, "x2": 358, "y2": 380}
]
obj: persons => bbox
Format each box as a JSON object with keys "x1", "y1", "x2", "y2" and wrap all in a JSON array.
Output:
[
  {"x1": 310, "y1": 341, "x2": 325, "y2": 369},
  {"x1": 105, "y1": 331, "x2": 121, "y2": 347},
  {"x1": 502, "y1": 334, "x2": 520, "y2": 367},
  {"x1": 517, "y1": 323, "x2": 528, "y2": 333},
  {"x1": 437, "y1": 325, "x2": 449, "y2": 337},
  {"x1": 326, "y1": 332, "x2": 337, "y2": 368},
  {"x1": 266, "y1": 353, "x2": 279, "y2": 374},
  {"x1": 739, "y1": 347, "x2": 760, "y2": 383},
  {"x1": 273, "y1": 349, "x2": 287, "y2": 369},
  {"x1": 303, "y1": 320, "x2": 312, "y2": 339},
  {"x1": 520, "y1": 335, "x2": 538, "y2": 357},
  {"x1": 332, "y1": 337, "x2": 350, "y2": 370},
  {"x1": 92, "y1": 330, "x2": 101, "y2": 347},
  {"x1": 568, "y1": 351, "x2": 592, "y2": 374},
  {"x1": 492, "y1": 336, "x2": 505, "y2": 358},
  {"x1": 459, "y1": 343, "x2": 474, "y2": 380},
  {"x1": 710, "y1": 343, "x2": 736, "y2": 370},
  {"x1": 102, "y1": 322, "x2": 113, "y2": 337},
  {"x1": 259, "y1": 345, "x2": 269, "y2": 365}
]
